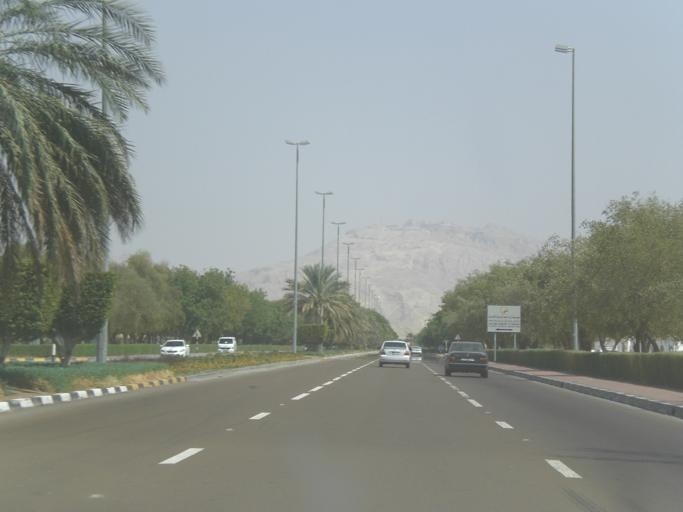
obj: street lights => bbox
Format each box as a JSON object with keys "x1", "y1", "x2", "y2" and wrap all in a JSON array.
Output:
[
  {"x1": 330, "y1": 221, "x2": 345, "y2": 276},
  {"x1": 343, "y1": 241, "x2": 371, "y2": 308},
  {"x1": 283, "y1": 139, "x2": 312, "y2": 354},
  {"x1": 315, "y1": 189, "x2": 333, "y2": 265}
]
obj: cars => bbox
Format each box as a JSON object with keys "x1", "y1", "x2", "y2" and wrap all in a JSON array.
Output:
[
  {"x1": 218, "y1": 336, "x2": 237, "y2": 353},
  {"x1": 378, "y1": 340, "x2": 412, "y2": 369},
  {"x1": 445, "y1": 340, "x2": 489, "y2": 379},
  {"x1": 409, "y1": 344, "x2": 422, "y2": 354},
  {"x1": 160, "y1": 339, "x2": 190, "y2": 358}
]
